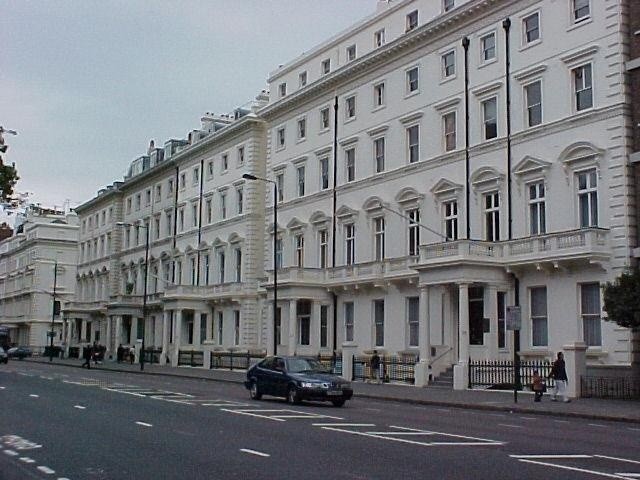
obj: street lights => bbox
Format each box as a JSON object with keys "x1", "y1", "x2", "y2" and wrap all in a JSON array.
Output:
[
  {"x1": 112, "y1": 219, "x2": 151, "y2": 372},
  {"x1": 241, "y1": 170, "x2": 280, "y2": 354},
  {"x1": 31, "y1": 256, "x2": 59, "y2": 362}
]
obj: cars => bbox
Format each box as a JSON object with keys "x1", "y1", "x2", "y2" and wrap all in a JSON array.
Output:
[
  {"x1": 7, "y1": 347, "x2": 34, "y2": 359},
  {"x1": 243, "y1": 351, "x2": 354, "y2": 409},
  {"x1": 0, "y1": 347, "x2": 9, "y2": 364}
]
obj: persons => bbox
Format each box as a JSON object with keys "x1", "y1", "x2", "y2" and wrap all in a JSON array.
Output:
[
  {"x1": 118, "y1": 343, "x2": 125, "y2": 361},
  {"x1": 532, "y1": 371, "x2": 545, "y2": 402},
  {"x1": 129, "y1": 345, "x2": 136, "y2": 365},
  {"x1": 548, "y1": 350, "x2": 571, "y2": 404},
  {"x1": 81, "y1": 345, "x2": 91, "y2": 369},
  {"x1": 91, "y1": 340, "x2": 106, "y2": 365},
  {"x1": 369, "y1": 350, "x2": 382, "y2": 385}
]
